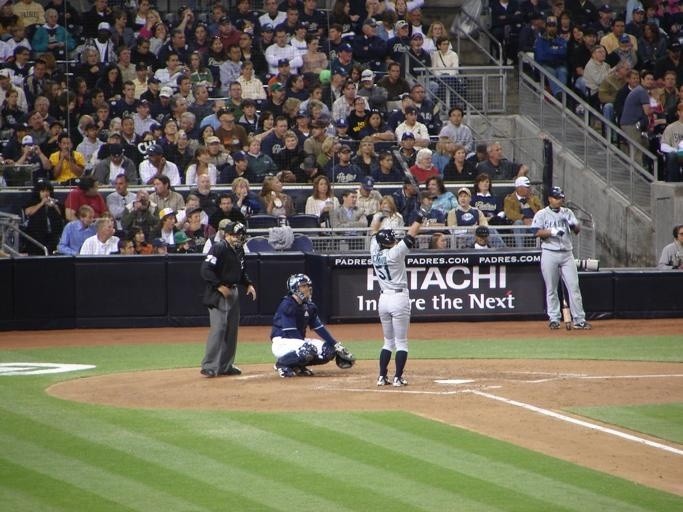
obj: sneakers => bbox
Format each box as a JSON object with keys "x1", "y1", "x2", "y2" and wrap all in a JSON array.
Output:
[
  {"x1": 271, "y1": 366, "x2": 312, "y2": 376},
  {"x1": 223, "y1": 367, "x2": 240, "y2": 375},
  {"x1": 549, "y1": 323, "x2": 560, "y2": 328},
  {"x1": 376, "y1": 376, "x2": 408, "y2": 387},
  {"x1": 573, "y1": 322, "x2": 592, "y2": 330}
]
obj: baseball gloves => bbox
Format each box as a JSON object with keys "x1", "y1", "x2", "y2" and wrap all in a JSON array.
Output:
[{"x1": 334, "y1": 341, "x2": 356, "y2": 369}]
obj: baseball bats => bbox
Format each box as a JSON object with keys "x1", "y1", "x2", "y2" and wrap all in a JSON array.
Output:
[
  {"x1": 558, "y1": 266, "x2": 572, "y2": 330},
  {"x1": 393, "y1": 149, "x2": 423, "y2": 204}
]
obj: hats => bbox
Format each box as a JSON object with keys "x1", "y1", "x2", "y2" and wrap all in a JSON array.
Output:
[
  {"x1": 337, "y1": 118, "x2": 347, "y2": 127},
  {"x1": 397, "y1": 20, "x2": 408, "y2": 30},
  {"x1": 402, "y1": 132, "x2": 414, "y2": 141},
  {"x1": 337, "y1": 43, "x2": 354, "y2": 51},
  {"x1": 516, "y1": 176, "x2": 531, "y2": 188},
  {"x1": 218, "y1": 106, "x2": 233, "y2": 118},
  {"x1": 362, "y1": 176, "x2": 373, "y2": 189},
  {"x1": 632, "y1": 6, "x2": 644, "y2": 13},
  {"x1": 278, "y1": 59, "x2": 289, "y2": 66},
  {"x1": 418, "y1": 191, "x2": 438, "y2": 200},
  {"x1": 150, "y1": 207, "x2": 201, "y2": 248},
  {"x1": 235, "y1": 151, "x2": 248, "y2": 161},
  {"x1": 15, "y1": 122, "x2": 36, "y2": 146},
  {"x1": 147, "y1": 145, "x2": 164, "y2": 155},
  {"x1": 100, "y1": 22, "x2": 108, "y2": 31},
  {"x1": 600, "y1": 3, "x2": 612, "y2": 13},
  {"x1": 218, "y1": 15, "x2": 229, "y2": 24},
  {"x1": 309, "y1": 119, "x2": 328, "y2": 128},
  {"x1": 361, "y1": 68, "x2": 373, "y2": 82},
  {"x1": 363, "y1": 18, "x2": 378, "y2": 27},
  {"x1": 206, "y1": 137, "x2": 220, "y2": 144},
  {"x1": 410, "y1": 32, "x2": 422, "y2": 40},
  {"x1": 457, "y1": 187, "x2": 471, "y2": 195},
  {"x1": 135, "y1": 61, "x2": 172, "y2": 105},
  {"x1": 271, "y1": 82, "x2": 284, "y2": 91}
]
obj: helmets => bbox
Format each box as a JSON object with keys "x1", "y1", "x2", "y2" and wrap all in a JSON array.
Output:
[
  {"x1": 377, "y1": 229, "x2": 396, "y2": 250},
  {"x1": 550, "y1": 186, "x2": 564, "y2": 199},
  {"x1": 287, "y1": 273, "x2": 313, "y2": 304},
  {"x1": 619, "y1": 33, "x2": 630, "y2": 42},
  {"x1": 225, "y1": 221, "x2": 247, "y2": 270}
]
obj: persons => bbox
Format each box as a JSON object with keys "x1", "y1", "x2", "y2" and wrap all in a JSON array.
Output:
[
  {"x1": 531, "y1": 186, "x2": 592, "y2": 330},
  {"x1": 660, "y1": 225, "x2": 683, "y2": 269},
  {"x1": 1, "y1": 2, "x2": 541, "y2": 257},
  {"x1": 270, "y1": 273, "x2": 355, "y2": 378},
  {"x1": 490, "y1": 1, "x2": 683, "y2": 182},
  {"x1": 199, "y1": 221, "x2": 257, "y2": 376},
  {"x1": 369, "y1": 207, "x2": 428, "y2": 387}
]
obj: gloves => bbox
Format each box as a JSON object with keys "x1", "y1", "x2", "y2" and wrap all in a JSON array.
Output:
[{"x1": 419, "y1": 204, "x2": 432, "y2": 216}]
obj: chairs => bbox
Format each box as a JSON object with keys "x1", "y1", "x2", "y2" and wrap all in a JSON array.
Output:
[
  {"x1": 0, "y1": 9, "x2": 392, "y2": 187},
  {"x1": 227, "y1": 191, "x2": 444, "y2": 254},
  {"x1": 565, "y1": 69, "x2": 669, "y2": 180}
]
